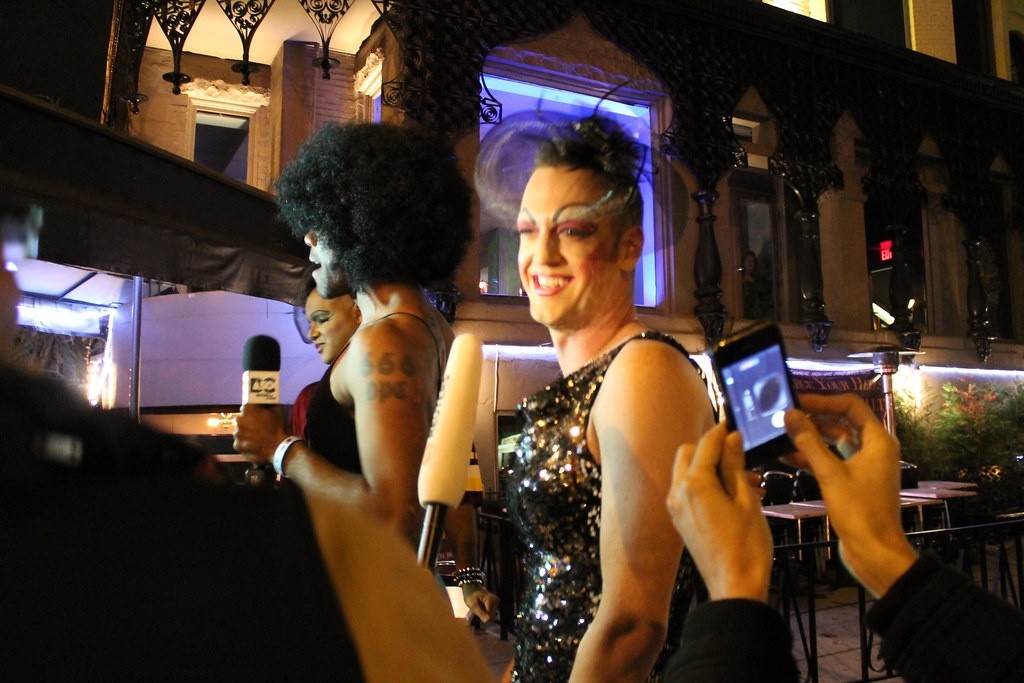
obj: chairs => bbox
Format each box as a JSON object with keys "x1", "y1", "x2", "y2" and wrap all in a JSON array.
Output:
[{"x1": 764, "y1": 467, "x2": 831, "y2": 583}]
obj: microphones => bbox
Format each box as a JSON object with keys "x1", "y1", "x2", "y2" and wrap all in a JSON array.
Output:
[
  {"x1": 237, "y1": 333, "x2": 281, "y2": 472},
  {"x1": 416, "y1": 331, "x2": 484, "y2": 575}
]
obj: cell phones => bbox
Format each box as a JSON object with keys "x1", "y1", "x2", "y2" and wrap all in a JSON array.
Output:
[{"x1": 710, "y1": 322, "x2": 802, "y2": 471}]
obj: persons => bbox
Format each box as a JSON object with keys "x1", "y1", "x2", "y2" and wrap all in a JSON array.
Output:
[
  {"x1": 0, "y1": 261, "x2": 261, "y2": 683},
  {"x1": 662, "y1": 390, "x2": 1024, "y2": 682},
  {"x1": 231, "y1": 116, "x2": 719, "y2": 683}
]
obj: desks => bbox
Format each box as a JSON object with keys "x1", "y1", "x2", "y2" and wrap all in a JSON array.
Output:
[{"x1": 760, "y1": 480, "x2": 981, "y2": 590}]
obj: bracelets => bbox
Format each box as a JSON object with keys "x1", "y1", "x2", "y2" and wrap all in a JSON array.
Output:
[
  {"x1": 452, "y1": 567, "x2": 486, "y2": 587},
  {"x1": 273, "y1": 434, "x2": 305, "y2": 478}
]
